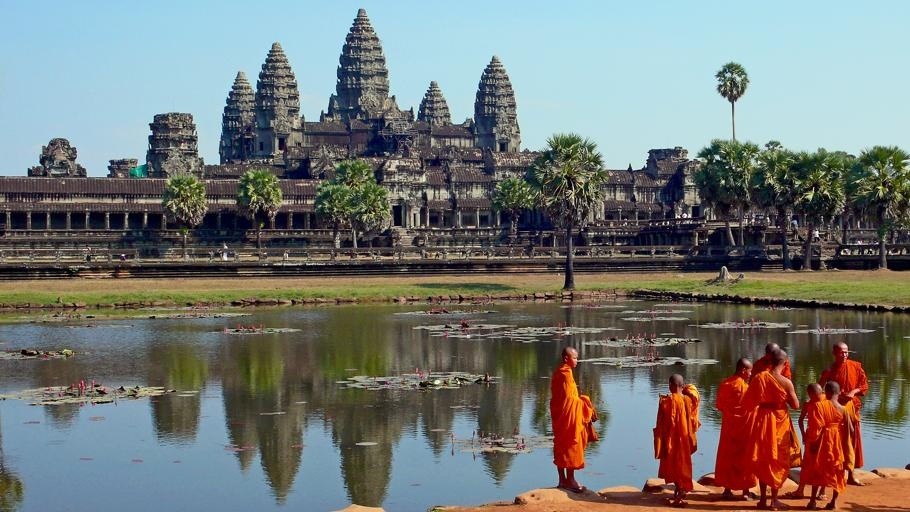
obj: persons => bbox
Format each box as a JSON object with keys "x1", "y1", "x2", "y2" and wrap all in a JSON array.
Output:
[
  {"x1": 813, "y1": 229, "x2": 820, "y2": 243},
  {"x1": 652, "y1": 372, "x2": 701, "y2": 503},
  {"x1": 549, "y1": 344, "x2": 599, "y2": 494},
  {"x1": 715, "y1": 357, "x2": 757, "y2": 500},
  {"x1": 840, "y1": 239, "x2": 879, "y2": 255},
  {"x1": 818, "y1": 341, "x2": 869, "y2": 486},
  {"x1": 751, "y1": 343, "x2": 793, "y2": 383},
  {"x1": 741, "y1": 348, "x2": 800, "y2": 511},
  {"x1": 784, "y1": 382, "x2": 828, "y2": 499},
  {"x1": 85, "y1": 244, "x2": 92, "y2": 263},
  {"x1": 806, "y1": 380, "x2": 856, "y2": 510},
  {"x1": 222, "y1": 242, "x2": 229, "y2": 261}
]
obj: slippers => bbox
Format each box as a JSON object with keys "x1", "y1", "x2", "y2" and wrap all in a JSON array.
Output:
[
  {"x1": 847, "y1": 478, "x2": 865, "y2": 486},
  {"x1": 757, "y1": 492, "x2": 836, "y2": 511},
  {"x1": 673, "y1": 500, "x2": 688, "y2": 507},
  {"x1": 721, "y1": 492, "x2": 757, "y2": 501}
]
